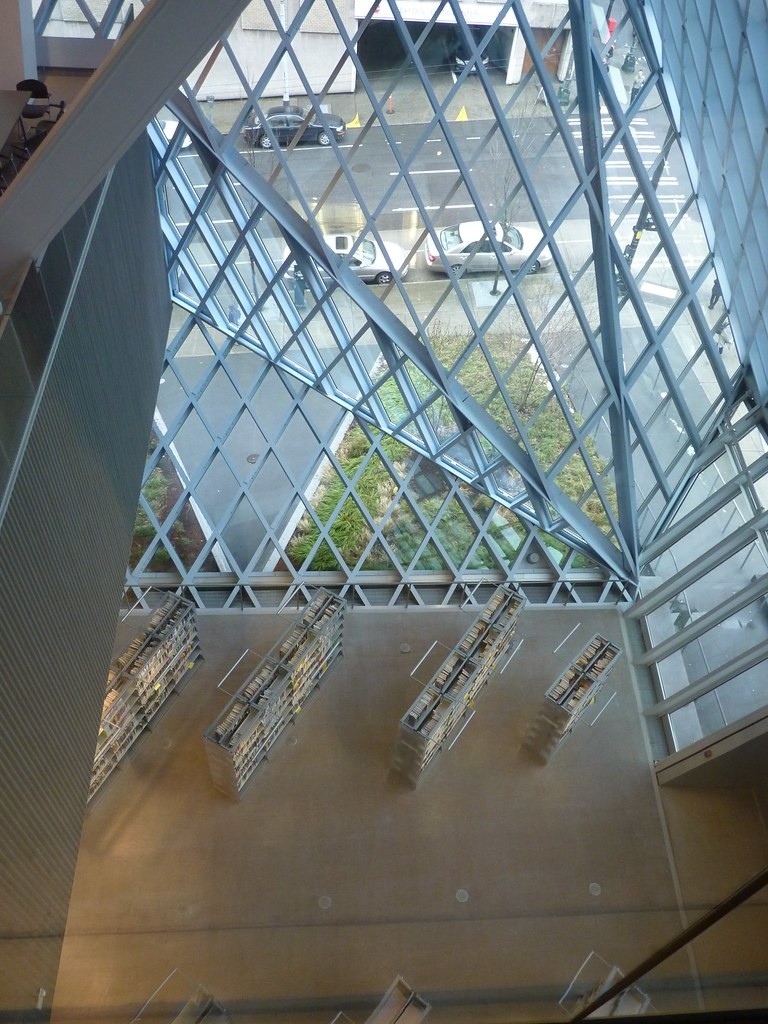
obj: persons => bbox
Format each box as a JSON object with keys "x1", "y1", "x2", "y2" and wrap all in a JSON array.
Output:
[
  {"x1": 631, "y1": 70, "x2": 644, "y2": 104},
  {"x1": 717, "y1": 320, "x2": 733, "y2": 354},
  {"x1": 708, "y1": 279, "x2": 722, "y2": 310}
]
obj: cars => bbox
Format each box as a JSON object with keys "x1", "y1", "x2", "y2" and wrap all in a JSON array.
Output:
[
  {"x1": 282, "y1": 234, "x2": 410, "y2": 288},
  {"x1": 425, "y1": 220, "x2": 553, "y2": 279},
  {"x1": 158, "y1": 120, "x2": 192, "y2": 149},
  {"x1": 446, "y1": 24, "x2": 489, "y2": 75},
  {"x1": 243, "y1": 105, "x2": 347, "y2": 149}
]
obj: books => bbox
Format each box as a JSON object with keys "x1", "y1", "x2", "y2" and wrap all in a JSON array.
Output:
[
  {"x1": 524, "y1": 634, "x2": 619, "y2": 757},
  {"x1": 87, "y1": 598, "x2": 198, "y2": 802},
  {"x1": 206, "y1": 594, "x2": 343, "y2": 792},
  {"x1": 392, "y1": 591, "x2": 523, "y2": 782}
]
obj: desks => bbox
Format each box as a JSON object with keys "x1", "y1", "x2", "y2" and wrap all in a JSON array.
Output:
[{"x1": 0, "y1": 89, "x2": 32, "y2": 152}]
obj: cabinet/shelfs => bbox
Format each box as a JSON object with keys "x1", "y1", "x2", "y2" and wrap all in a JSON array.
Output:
[
  {"x1": 202, "y1": 586, "x2": 347, "y2": 804},
  {"x1": 87, "y1": 590, "x2": 207, "y2": 815},
  {"x1": 388, "y1": 584, "x2": 527, "y2": 791},
  {"x1": 520, "y1": 631, "x2": 623, "y2": 768}
]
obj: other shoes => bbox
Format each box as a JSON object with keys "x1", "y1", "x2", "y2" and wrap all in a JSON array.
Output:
[{"x1": 708, "y1": 306, "x2": 714, "y2": 309}]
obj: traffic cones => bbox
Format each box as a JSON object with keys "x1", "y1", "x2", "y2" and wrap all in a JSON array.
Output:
[{"x1": 386, "y1": 96, "x2": 394, "y2": 114}]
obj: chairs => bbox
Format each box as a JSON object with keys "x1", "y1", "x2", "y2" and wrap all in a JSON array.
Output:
[
  {"x1": 16, "y1": 79, "x2": 52, "y2": 141},
  {"x1": 27, "y1": 101, "x2": 66, "y2": 135}
]
